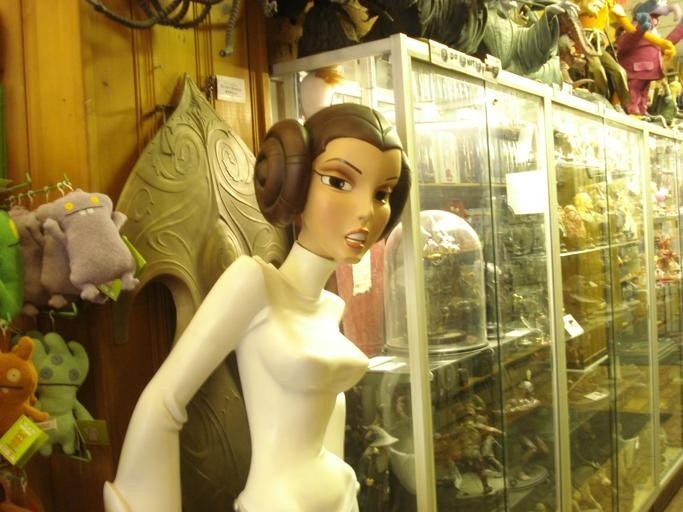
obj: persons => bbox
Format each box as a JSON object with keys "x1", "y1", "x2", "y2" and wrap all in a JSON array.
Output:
[
  {"x1": 102, "y1": 102, "x2": 413, "y2": 512},
  {"x1": 562, "y1": 0, "x2": 683, "y2": 118}
]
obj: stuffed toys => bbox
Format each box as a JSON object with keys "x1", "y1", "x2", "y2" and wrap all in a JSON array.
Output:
[{"x1": 0, "y1": 188, "x2": 141, "y2": 462}]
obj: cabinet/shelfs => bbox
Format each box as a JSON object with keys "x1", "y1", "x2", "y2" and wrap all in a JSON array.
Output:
[{"x1": 270, "y1": 33, "x2": 683, "y2": 511}]
